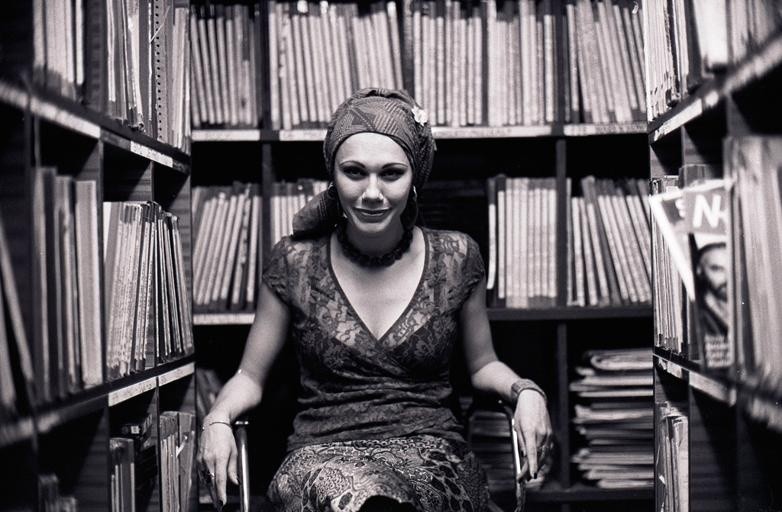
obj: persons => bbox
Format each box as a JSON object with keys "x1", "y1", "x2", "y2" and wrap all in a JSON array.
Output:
[{"x1": 196, "y1": 87, "x2": 553, "y2": 512}]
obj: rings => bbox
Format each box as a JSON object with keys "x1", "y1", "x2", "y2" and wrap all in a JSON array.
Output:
[{"x1": 208, "y1": 472, "x2": 217, "y2": 479}]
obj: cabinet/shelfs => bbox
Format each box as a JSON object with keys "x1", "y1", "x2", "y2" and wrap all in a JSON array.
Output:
[{"x1": 0, "y1": 0, "x2": 782, "y2": 512}]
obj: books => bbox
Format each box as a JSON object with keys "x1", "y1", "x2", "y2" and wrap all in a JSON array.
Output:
[
  {"x1": 1, "y1": 2, "x2": 190, "y2": 156},
  {"x1": 409, "y1": 2, "x2": 554, "y2": 128},
  {"x1": 642, "y1": 4, "x2": 781, "y2": 512},
  {"x1": 6, "y1": 410, "x2": 194, "y2": 512},
  {"x1": 194, "y1": 353, "x2": 224, "y2": 503},
  {"x1": 564, "y1": 2, "x2": 648, "y2": 127},
  {"x1": 186, "y1": 2, "x2": 262, "y2": 129},
  {"x1": 569, "y1": 346, "x2": 655, "y2": 490},
  {"x1": 456, "y1": 389, "x2": 550, "y2": 491},
  {"x1": 2, "y1": 164, "x2": 194, "y2": 415},
  {"x1": 188, "y1": 182, "x2": 327, "y2": 316},
  {"x1": 566, "y1": 176, "x2": 654, "y2": 310},
  {"x1": 483, "y1": 173, "x2": 556, "y2": 310},
  {"x1": 275, "y1": 3, "x2": 405, "y2": 131}
]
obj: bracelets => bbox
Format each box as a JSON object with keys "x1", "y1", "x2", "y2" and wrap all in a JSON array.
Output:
[
  {"x1": 201, "y1": 421, "x2": 232, "y2": 432},
  {"x1": 510, "y1": 380, "x2": 547, "y2": 408}
]
